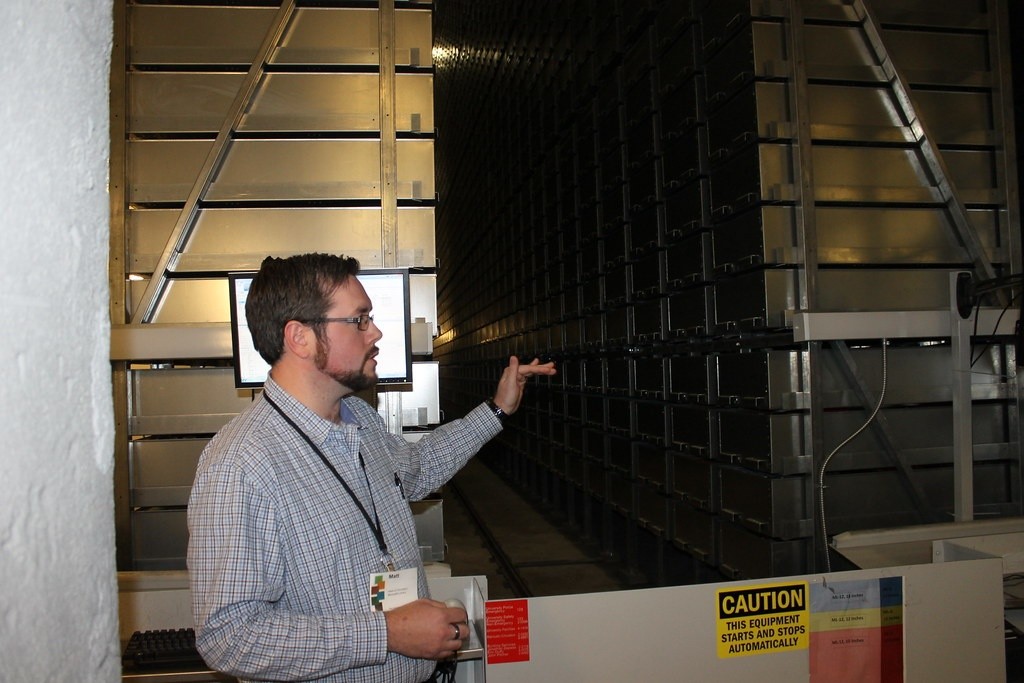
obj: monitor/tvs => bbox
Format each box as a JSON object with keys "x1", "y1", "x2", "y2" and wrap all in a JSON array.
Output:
[{"x1": 228, "y1": 268, "x2": 413, "y2": 390}]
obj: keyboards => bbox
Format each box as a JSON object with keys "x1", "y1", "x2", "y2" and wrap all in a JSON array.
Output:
[{"x1": 122, "y1": 627, "x2": 205, "y2": 668}]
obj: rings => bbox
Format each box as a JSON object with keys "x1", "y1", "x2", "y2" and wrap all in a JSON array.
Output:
[{"x1": 451, "y1": 623, "x2": 460, "y2": 640}]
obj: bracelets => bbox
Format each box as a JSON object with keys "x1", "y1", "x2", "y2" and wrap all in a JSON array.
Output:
[{"x1": 485, "y1": 396, "x2": 508, "y2": 422}]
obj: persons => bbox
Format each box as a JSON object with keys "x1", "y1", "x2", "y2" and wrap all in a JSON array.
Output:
[{"x1": 188, "y1": 251, "x2": 556, "y2": 683}]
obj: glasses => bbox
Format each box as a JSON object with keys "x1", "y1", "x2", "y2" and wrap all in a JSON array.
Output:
[{"x1": 294, "y1": 314, "x2": 373, "y2": 331}]
